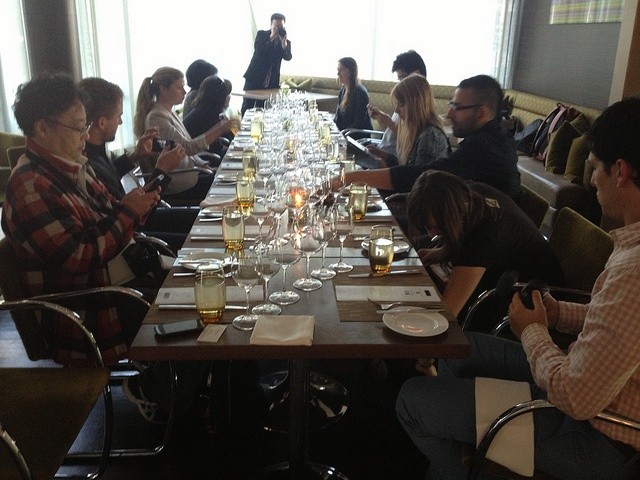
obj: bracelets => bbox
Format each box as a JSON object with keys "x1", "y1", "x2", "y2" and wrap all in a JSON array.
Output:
[{"x1": 416, "y1": 358, "x2": 435, "y2": 369}]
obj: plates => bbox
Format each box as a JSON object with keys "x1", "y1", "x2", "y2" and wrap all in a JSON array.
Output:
[
  {"x1": 199, "y1": 206, "x2": 233, "y2": 217},
  {"x1": 215, "y1": 108, "x2": 258, "y2": 186},
  {"x1": 382, "y1": 306, "x2": 449, "y2": 336},
  {"x1": 183, "y1": 251, "x2": 233, "y2": 272},
  {"x1": 362, "y1": 237, "x2": 410, "y2": 255}
]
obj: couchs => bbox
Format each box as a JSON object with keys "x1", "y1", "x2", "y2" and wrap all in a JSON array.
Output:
[
  {"x1": 0, "y1": 131, "x2": 26, "y2": 208},
  {"x1": 283, "y1": 73, "x2": 603, "y2": 206}
]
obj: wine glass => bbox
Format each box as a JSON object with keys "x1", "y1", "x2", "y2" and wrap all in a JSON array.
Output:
[
  {"x1": 329, "y1": 204, "x2": 353, "y2": 273},
  {"x1": 259, "y1": 88, "x2": 339, "y2": 172},
  {"x1": 310, "y1": 209, "x2": 336, "y2": 280},
  {"x1": 293, "y1": 218, "x2": 323, "y2": 292},
  {"x1": 251, "y1": 245, "x2": 282, "y2": 315},
  {"x1": 250, "y1": 172, "x2": 291, "y2": 250},
  {"x1": 291, "y1": 138, "x2": 345, "y2": 218},
  {"x1": 231, "y1": 250, "x2": 263, "y2": 332},
  {"x1": 268, "y1": 231, "x2": 302, "y2": 306}
]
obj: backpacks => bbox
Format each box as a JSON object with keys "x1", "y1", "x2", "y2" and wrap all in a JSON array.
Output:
[{"x1": 530, "y1": 104, "x2": 581, "y2": 161}]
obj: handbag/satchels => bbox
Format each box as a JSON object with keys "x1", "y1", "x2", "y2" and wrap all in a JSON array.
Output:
[{"x1": 514, "y1": 118, "x2": 548, "y2": 156}]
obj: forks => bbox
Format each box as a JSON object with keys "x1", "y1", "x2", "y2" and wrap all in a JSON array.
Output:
[{"x1": 369, "y1": 298, "x2": 439, "y2": 309}]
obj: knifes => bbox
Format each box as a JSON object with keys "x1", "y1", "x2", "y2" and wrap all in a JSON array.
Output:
[
  {"x1": 190, "y1": 236, "x2": 257, "y2": 242},
  {"x1": 373, "y1": 307, "x2": 445, "y2": 313},
  {"x1": 159, "y1": 303, "x2": 246, "y2": 312},
  {"x1": 349, "y1": 269, "x2": 423, "y2": 279}
]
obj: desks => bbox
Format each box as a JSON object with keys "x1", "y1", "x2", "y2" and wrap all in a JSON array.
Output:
[{"x1": 232, "y1": 86, "x2": 341, "y2": 107}]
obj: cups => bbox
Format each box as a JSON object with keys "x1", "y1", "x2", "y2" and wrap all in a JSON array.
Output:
[
  {"x1": 349, "y1": 180, "x2": 368, "y2": 220},
  {"x1": 368, "y1": 224, "x2": 395, "y2": 273},
  {"x1": 242, "y1": 150, "x2": 258, "y2": 175},
  {"x1": 250, "y1": 119, "x2": 262, "y2": 140},
  {"x1": 222, "y1": 205, "x2": 244, "y2": 251},
  {"x1": 340, "y1": 152, "x2": 355, "y2": 175},
  {"x1": 195, "y1": 263, "x2": 226, "y2": 323},
  {"x1": 236, "y1": 172, "x2": 255, "y2": 206}
]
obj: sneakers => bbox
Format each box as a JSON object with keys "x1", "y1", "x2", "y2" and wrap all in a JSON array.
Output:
[{"x1": 122, "y1": 375, "x2": 161, "y2": 424}]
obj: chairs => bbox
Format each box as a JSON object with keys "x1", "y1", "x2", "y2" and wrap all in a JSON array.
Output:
[
  {"x1": 134, "y1": 151, "x2": 218, "y2": 206},
  {"x1": 0, "y1": 229, "x2": 177, "y2": 368},
  {"x1": 218, "y1": 135, "x2": 234, "y2": 147},
  {"x1": 457, "y1": 208, "x2": 615, "y2": 335},
  {"x1": 466, "y1": 283, "x2": 640, "y2": 480},
  {"x1": 0, "y1": 300, "x2": 166, "y2": 480},
  {"x1": 342, "y1": 130, "x2": 387, "y2": 169}
]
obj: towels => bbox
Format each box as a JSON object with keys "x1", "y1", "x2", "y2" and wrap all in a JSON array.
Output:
[{"x1": 474, "y1": 375, "x2": 535, "y2": 477}]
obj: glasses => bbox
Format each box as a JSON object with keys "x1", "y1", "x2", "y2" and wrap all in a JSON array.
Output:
[
  {"x1": 447, "y1": 102, "x2": 484, "y2": 111},
  {"x1": 40, "y1": 119, "x2": 93, "y2": 135},
  {"x1": 272, "y1": 23, "x2": 283, "y2": 28},
  {"x1": 397, "y1": 75, "x2": 409, "y2": 80}
]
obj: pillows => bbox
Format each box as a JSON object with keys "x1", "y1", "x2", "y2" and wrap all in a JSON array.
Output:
[
  {"x1": 571, "y1": 113, "x2": 589, "y2": 135},
  {"x1": 544, "y1": 120, "x2": 581, "y2": 173},
  {"x1": 563, "y1": 134, "x2": 590, "y2": 184}
]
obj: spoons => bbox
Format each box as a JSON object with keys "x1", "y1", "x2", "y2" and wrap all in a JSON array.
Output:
[
  {"x1": 198, "y1": 210, "x2": 244, "y2": 223},
  {"x1": 172, "y1": 264, "x2": 240, "y2": 279}
]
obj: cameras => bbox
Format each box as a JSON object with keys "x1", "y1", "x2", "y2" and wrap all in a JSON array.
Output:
[
  {"x1": 275, "y1": 26, "x2": 287, "y2": 37},
  {"x1": 520, "y1": 277, "x2": 551, "y2": 309}
]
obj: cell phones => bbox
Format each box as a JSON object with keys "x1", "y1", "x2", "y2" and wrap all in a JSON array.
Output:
[
  {"x1": 153, "y1": 317, "x2": 205, "y2": 336},
  {"x1": 148, "y1": 175, "x2": 166, "y2": 191},
  {"x1": 152, "y1": 138, "x2": 174, "y2": 153}
]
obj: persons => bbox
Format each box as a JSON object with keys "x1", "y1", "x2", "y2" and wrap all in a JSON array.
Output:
[
  {"x1": 134, "y1": 66, "x2": 241, "y2": 199},
  {"x1": 182, "y1": 60, "x2": 218, "y2": 119},
  {"x1": 241, "y1": 12, "x2": 292, "y2": 117},
  {"x1": 366, "y1": 50, "x2": 427, "y2": 153},
  {"x1": 398, "y1": 97, "x2": 639, "y2": 480},
  {"x1": 77, "y1": 77, "x2": 186, "y2": 192},
  {"x1": 333, "y1": 57, "x2": 373, "y2": 140},
  {"x1": 182, "y1": 75, "x2": 232, "y2": 156},
  {"x1": 2, "y1": 71, "x2": 163, "y2": 423},
  {"x1": 315, "y1": 74, "x2": 520, "y2": 205},
  {"x1": 407, "y1": 170, "x2": 559, "y2": 377},
  {"x1": 367, "y1": 73, "x2": 451, "y2": 170}
]
